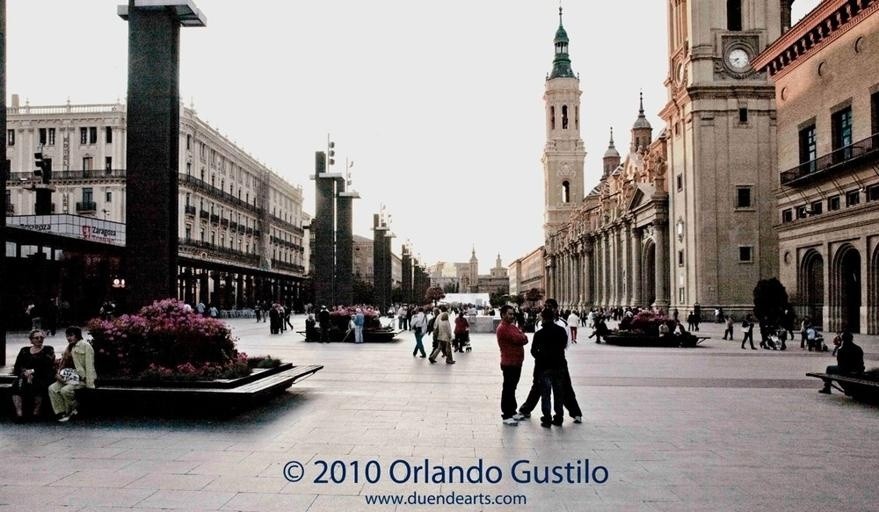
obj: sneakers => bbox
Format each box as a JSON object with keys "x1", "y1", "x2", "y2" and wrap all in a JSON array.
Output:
[
  {"x1": 413, "y1": 343, "x2": 466, "y2": 364},
  {"x1": 503, "y1": 411, "x2": 582, "y2": 428}
]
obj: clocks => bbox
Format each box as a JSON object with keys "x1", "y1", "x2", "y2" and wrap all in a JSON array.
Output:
[{"x1": 729, "y1": 47, "x2": 749, "y2": 68}]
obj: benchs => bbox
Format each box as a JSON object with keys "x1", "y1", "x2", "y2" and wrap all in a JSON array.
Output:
[
  {"x1": 295, "y1": 326, "x2": 405, "y2": 341},
  {"x1": 806, "y1": 370, "x2": 878, "y2": 399}
]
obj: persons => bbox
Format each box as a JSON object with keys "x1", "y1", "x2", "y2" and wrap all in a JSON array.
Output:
[
  {"x1": 530, "y1": 309, "x2": 568, "y2": 429},
  {"x1": 512, "y1": 297, "x2": 583, "y2": 424},
  {"x1": 831, "y1": 331, "x2": 843, "y2": 356},
  {"x1": 818, "y1": 331, "x2": 865, "y2": 397},
  {"x1": 47, "y1": 324, "x2": 98, "y2": 422},
  {"x1": 496, "y1": 305, "x2": 529, "y2": 426},
  {"x1": 9, "y1": 329, "x2": 56, "y2": 424},
  {"x1": 0, "y1": 291, "x2": 829, "y2": 365}
]
